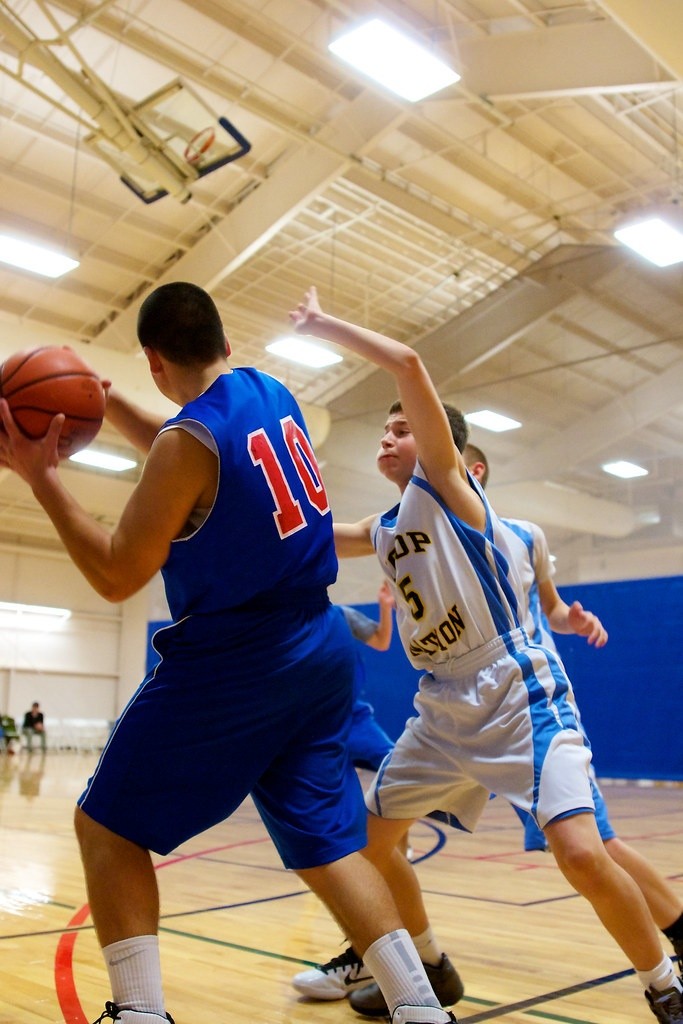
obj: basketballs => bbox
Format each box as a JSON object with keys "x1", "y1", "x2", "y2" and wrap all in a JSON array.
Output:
[{"x1": 0, "y1": 345, "x2": 109, "y2": 464}]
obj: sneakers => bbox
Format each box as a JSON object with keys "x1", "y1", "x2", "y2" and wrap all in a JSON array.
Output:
[
  {"x1": 290, "y1": 946, "x2": 376, "y2": 1001},
  {"x1": 93, "y1": 1001, "x2": 174, "y2": 1024},
  {"x1": 644, "y1": 977, "x2": 683, "y2": 1023},
  {"x1": 350, "y1": 951, "x2": 464, "y2": 1015},
  {"x1": 388, "y1": 1005, "x2": 457, "y2": 1024}
]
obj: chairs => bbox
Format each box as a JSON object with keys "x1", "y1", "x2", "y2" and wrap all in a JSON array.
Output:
[{"x1": 15, "y1": 717, "x2": 111, "y2": 754}]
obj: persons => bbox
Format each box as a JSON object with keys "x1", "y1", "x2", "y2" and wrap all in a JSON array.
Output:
[
  {"x1": 288, "y1": 285, "x2": 682, "y2": 1023},
  {"x1": 330, "y1": 578, "x2": 395, "y2": 771},
  {"x1": 0, "y1": 717, "x2": 14, "y2": 754},
  {"x1": 292, "y1": 443, "x2": 682, "y2": 1000},
  {"x1": 0, "y1": 282, "x2": 458, "y2": 1024},
  {"x1": 22, "y1": 703, "x2": 46, "y2": 750}
]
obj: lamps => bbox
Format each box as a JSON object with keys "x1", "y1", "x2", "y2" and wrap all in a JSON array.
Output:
[
  {"x1": 264, "y1": 214, "x2": 343, "y2": 369},
  {"x1": 0, "y1": 108, "x2": 81, "y2": 278},
  {"x1": 613, "y1": 91, "x2": 683, "y2": 269},
  {"x1": 327, "y1": 0, "x2": 464, "y2": 103}
]
